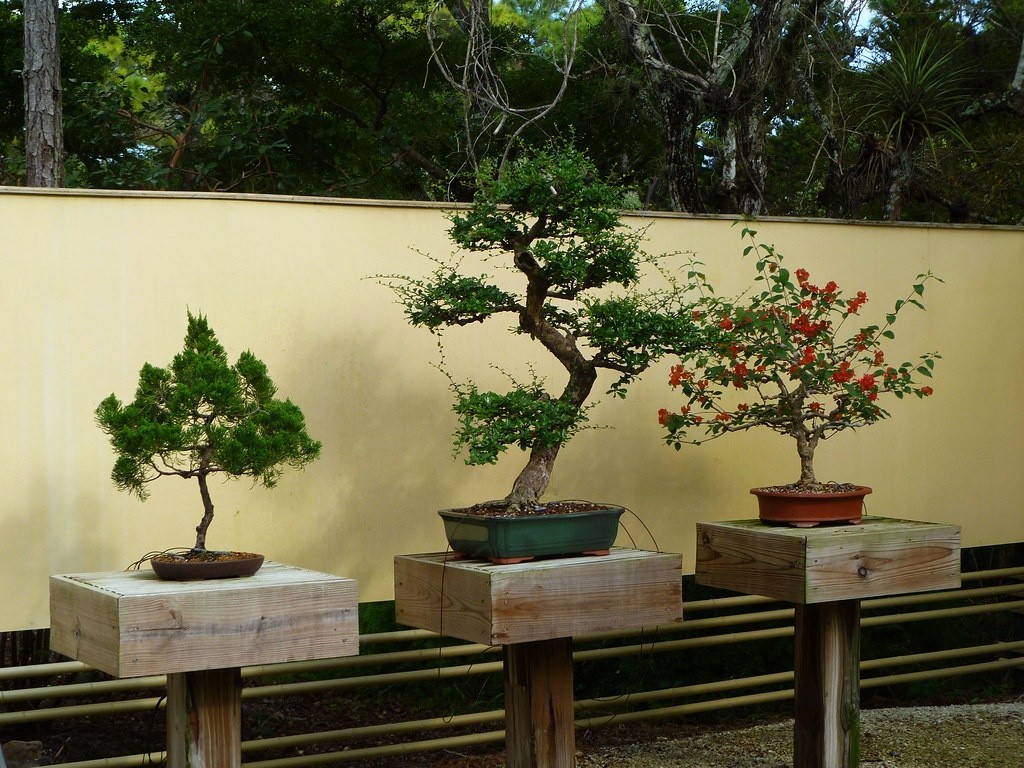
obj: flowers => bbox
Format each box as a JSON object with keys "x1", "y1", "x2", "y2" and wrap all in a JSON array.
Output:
[{"x1": 660, "y1": 208, "x2": 943, "y2": 490}]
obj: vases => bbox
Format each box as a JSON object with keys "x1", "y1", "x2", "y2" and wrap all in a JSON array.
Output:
[{"x1": 750, "y1": 484, "x2": 872, "y2": 531}]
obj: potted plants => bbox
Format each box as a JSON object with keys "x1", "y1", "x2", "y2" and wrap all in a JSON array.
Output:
[
  {"x1": 93, "y1": 305, "x2": 324, "y2": 581},
  {"x1": 358, "y1": 123, "x2": 757, "y2": 566}
]
